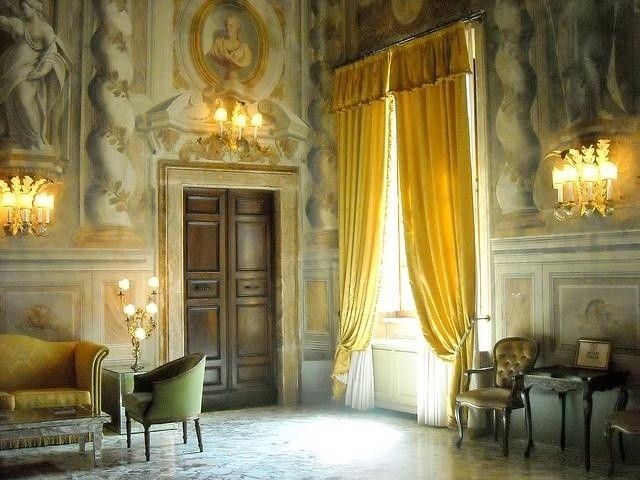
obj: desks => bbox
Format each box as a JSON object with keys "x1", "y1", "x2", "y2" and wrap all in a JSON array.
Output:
[
  {"x1": 102, "y1": 364, "x2": 178, "y2": 435},
  {"x1": 518, "y1": 365, "x2": 629, "y2": 472},
  {"x1": 0, "y1": 405, "x2": 114, "y2": 470}
]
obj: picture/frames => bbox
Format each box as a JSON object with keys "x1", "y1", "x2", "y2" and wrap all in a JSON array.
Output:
[{"x1": 573, "y1": 337, "x2": 614, "y2": 372}]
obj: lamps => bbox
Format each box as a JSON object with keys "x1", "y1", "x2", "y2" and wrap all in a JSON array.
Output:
[
  {"x1": 195, "y1": 99, "x2": 270, "y2": 161},
  {"x1": 549, "y1": 139, "x2": 620, "y2": 220},
  {"x1": 115, "y1": 276, "x2": 161, "y2": 370},
  {"x1": 0, "y1": 169, "x2": 59, "y2": 240}
]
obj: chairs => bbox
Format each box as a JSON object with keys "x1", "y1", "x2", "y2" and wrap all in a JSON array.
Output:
[
  {"x1": 128, "y1": 349, "x2": 209, "y2": 461},
  {"x1": 450, "y1": 336, "x2": 537, "y2": 457}
]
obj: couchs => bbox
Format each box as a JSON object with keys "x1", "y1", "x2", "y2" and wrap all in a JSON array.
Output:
[{"x1": 1, "y1": 329, "x2": 109, "y2": 411}]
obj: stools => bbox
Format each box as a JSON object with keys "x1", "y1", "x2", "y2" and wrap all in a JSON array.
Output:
[{"x1": 601, "y1": 411, "x2": 640, "y2": 479}]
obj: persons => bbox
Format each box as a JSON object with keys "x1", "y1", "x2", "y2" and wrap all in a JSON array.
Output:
[
  {"x1": 1, "y1": 0, "x2": 69, "y2": 155},
  {"x1": 210, "y1": 14, "x2": 253, "y2": 69}
]
